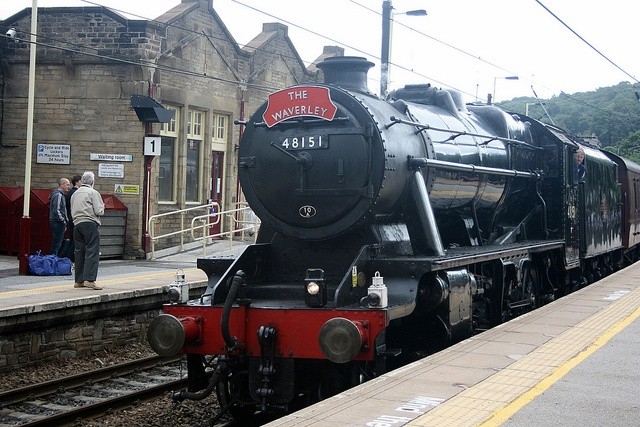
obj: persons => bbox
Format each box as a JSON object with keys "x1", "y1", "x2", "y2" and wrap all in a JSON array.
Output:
[
  {"x1": 65, "y1": 175, "x2": 83, "y2": 266},
  {"x1": 70, "y1": 171, "x2": 105, "y2": 290},
  {"x1": 49, "y1": 178, "x2": 70, "y2": 254}
]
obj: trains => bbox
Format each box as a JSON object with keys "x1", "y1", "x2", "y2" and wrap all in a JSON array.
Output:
[{"x1": 146, "y1": 82, "x2": 640, "y2": 418}]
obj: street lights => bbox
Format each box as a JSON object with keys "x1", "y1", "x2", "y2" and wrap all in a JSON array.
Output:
[
  {"x1": 525, "y1": 102, "x2": 547, "y2": 116},
  {"x1": 487, "y1": 76, "x2": 519, "y2": 105},
  {"x1": 380, "y1": 0, "x2": 429, "y2": 97}
]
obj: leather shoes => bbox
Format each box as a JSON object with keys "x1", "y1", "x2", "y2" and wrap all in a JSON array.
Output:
[
  {"x1": 84, "y1": 281, "x2": 103, "y2": 290},
  {"x1": 73, "y1": 282, "x2": 86, "y2": 288}
]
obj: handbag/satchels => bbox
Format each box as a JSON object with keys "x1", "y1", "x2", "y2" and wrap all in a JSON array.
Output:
[
  {"x1": 44, "y1": 253, "x2": 72, "y2": 275},
  {"x1": 28, "y1": 249, "x2": 56, "y2": 275}
]
